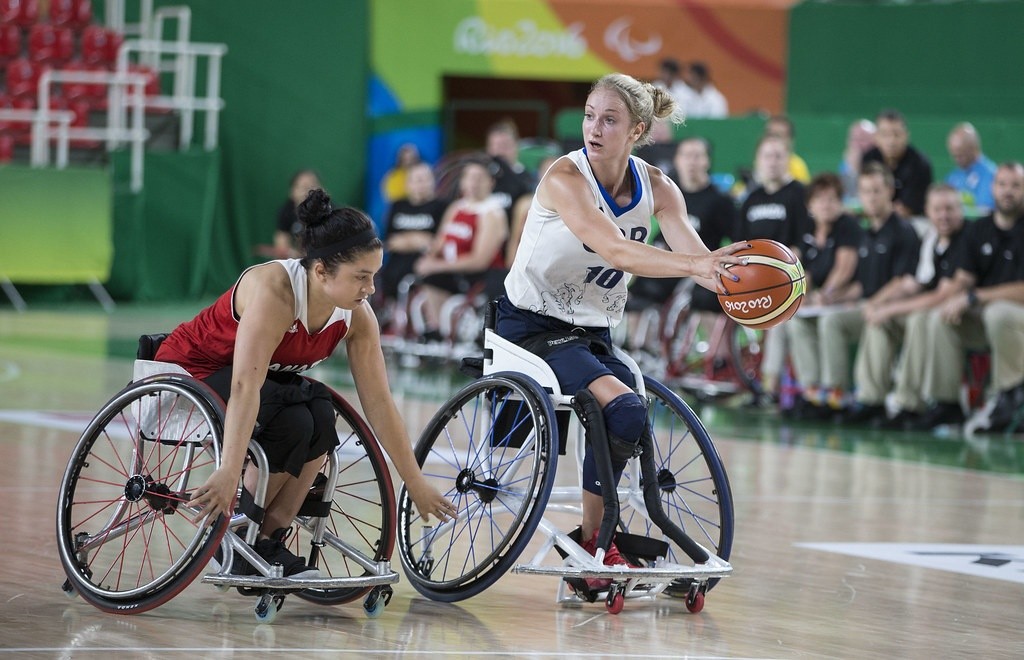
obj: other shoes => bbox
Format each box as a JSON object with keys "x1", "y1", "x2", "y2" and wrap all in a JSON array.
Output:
[
  {"x1": 377, "y1": 332, "x2": 484, "y2": 361},
  {"x1": 730, "y1": 389, "x2": 1024, "y2": 436},
  {"x1": 245, "y1": 526, "x2": 320, "y2": 593},
  {"x1": 213, "y1": 526, "x2": 259, "y2": 575}
]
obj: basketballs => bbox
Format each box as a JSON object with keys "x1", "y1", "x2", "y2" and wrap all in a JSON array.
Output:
[{"x1": 717, "y1": 239, "x2": 806, "y2": 329}]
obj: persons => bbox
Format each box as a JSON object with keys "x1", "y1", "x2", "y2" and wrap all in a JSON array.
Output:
[
  {"x1": 151, "y1": 181, "x2": 458, "y2": 580},
  {"x1": 485, "y1": 72, "x2": 753, "y2": 580},
  {"x1": 253, "y1": 60, "x2": 1024, "y2": 429}
]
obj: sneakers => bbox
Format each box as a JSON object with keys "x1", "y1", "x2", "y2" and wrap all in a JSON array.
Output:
[{"x1": 567, "y1": 528, "x2": 642, "y2": 591}]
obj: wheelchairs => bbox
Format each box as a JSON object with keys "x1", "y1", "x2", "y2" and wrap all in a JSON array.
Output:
[
  {"x1": 397, "y1": 296, "x2": 737, "y2": 613},
  {"x1": 375, "y1": 272, "x2": 761, "y2": 407},
  {"x1": 56, "y1": 333, "x2": 399, "y2": 625}
]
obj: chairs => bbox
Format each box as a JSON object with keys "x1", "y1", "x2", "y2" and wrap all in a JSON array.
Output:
[{"x1": 0, "y1": 0, "x2": 163, "y2": 158}]
